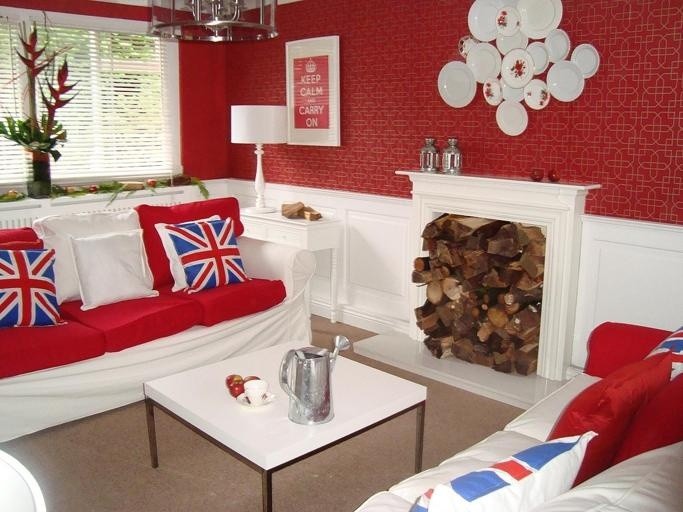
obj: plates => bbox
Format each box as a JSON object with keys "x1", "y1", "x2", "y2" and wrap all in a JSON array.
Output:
[{"x1": 237, "y1": 392, "x2": 273, "y2": 406}]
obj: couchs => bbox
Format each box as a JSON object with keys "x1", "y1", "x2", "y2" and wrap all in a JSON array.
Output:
[
  {"x1": 356, "y1": 321, "x2": 683, "y2": 512},
  {"x1": 0, "y1": 198, "x2": 315, "y2": 444}
]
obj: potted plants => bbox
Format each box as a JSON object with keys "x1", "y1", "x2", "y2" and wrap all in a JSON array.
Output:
[{"x1": 0, "y1": 28, "x2": 80, "y2": 202}]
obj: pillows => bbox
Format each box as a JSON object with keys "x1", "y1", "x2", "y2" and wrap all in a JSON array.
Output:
[
  {"x1": 411, "y1": 431, "x2": 599, "y2": 511},
  {"x1": 0, "y1": 249, "x2": 66, "y2": 328},
  {"x1": 547, "y1": 350, "x2": 672, "y2": 458},
  {"x1": 69, "y1": 227, "x2": 158, "y2": 312},
  {"x1": 643, "y1": 325, "x2": 682, "y2": 381},
  {"x1": 154, "y1": 215, "x2": 249, "y2": 295}
]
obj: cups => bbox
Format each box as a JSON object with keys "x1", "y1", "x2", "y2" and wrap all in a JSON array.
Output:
[{"x1": 239, "y1": 379, "x2": 267, "y2": 404}]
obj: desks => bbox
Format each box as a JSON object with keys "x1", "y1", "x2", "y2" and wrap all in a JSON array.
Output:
[{"x1": 239, "y1": 207, "x2": 341, "y2": 323}]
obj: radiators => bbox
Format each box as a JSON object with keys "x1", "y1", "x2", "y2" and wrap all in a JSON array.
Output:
[{"x1": 1, "y1": 183, "x2": 184, "y2": 228}]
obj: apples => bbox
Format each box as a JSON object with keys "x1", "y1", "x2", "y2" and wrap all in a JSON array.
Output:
[
  {"x1": 7, "y1": 190, "x2": 17, "y2": 198},
  {"x1": 146, "y1": 178, "x2": 156, "y2": 187},
  {"x1": 225, "y1": 374, "x2": 242, "y2": 389},
  {"x1": 530, "y1": 168, "x2": 544, "y2": 181},
  {"x1": 229, "y1": 380, "x2": 243, "y2": 398},
  {"x1": 243, "y1": 375, "x2": 260, "y2": 383},
  {"x1": 88, "y1": 184, "x2": 99, "y2": 192},
  {"x1": 547, "y1": 168, "x2": 561, "y2": 182}
]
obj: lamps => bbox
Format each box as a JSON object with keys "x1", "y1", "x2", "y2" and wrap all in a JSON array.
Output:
[
  {"x1": 149, "y1": 0, "x2": 277, "y2": 43},
  {"x1": 230, "y1": 107, "x2": 287, "y2": 217}
]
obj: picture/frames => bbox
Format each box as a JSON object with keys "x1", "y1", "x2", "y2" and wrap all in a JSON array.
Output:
[{"x1": 284, "y1": 36, "x2": 340, "y2": 146}]
